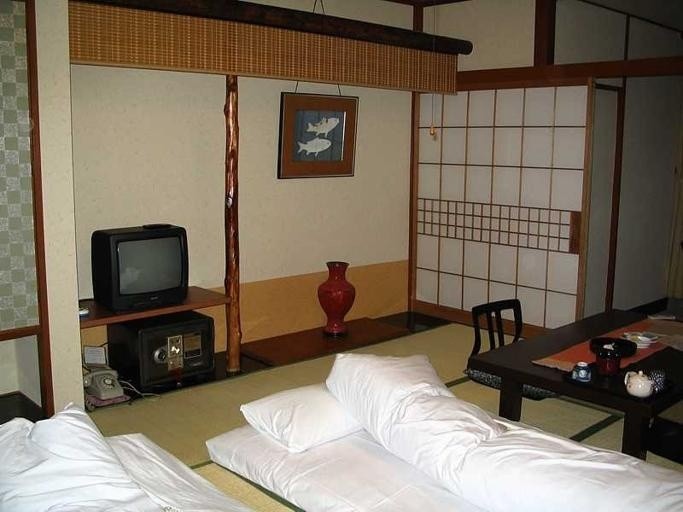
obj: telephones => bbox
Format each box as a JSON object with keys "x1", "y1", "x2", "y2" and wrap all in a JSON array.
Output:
[{"x1": 83, "y1": 369, "x2": 123, "y2": 401}]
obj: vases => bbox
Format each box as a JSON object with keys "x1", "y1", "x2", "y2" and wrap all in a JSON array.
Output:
[{"x1": 318, "y1": 262, "x2": 356, "y2": 336}]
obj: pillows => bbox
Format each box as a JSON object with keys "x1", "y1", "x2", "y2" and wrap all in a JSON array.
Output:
[
  {"x1": 0, "y1": 400, "x2": 149, "y2": 512},
  {"x1": 239, "y1": 381, "x2": 364, "y2": 455}
]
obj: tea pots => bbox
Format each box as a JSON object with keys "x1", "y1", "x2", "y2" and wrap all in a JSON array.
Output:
[{"x1": 624, "y1": 370, "x2": 655, "y2": 398}]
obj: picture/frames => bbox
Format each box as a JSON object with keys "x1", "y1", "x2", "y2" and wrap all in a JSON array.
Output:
[{"x1": 277, "y1": 91, "x2": 359, "y2": 180}]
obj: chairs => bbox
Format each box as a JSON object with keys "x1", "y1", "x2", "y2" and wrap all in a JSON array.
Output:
[{"x1": 463, "y1": 299, "x2": 554, "y2": 401}]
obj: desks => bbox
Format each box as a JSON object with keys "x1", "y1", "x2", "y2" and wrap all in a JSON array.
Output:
[{"x1": 468, "y1": 295, "x2": 683, "y2": 463}]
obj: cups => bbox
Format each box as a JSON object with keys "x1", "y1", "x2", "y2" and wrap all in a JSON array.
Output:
[
  {"x1": 572, "y1": 361, "x2": 592, "y2": 381},
  {"x1": 596, "y1": 352, "x2": 620, "y2": 376},
  {"x1": 649, "y1": 367, "x2": 666, "y2": 393}
]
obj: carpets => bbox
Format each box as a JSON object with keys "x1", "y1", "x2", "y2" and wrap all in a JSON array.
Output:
[
  {"x1": 190, "y1": 460, "x2": 307, "y2": 512},
  {"x1": 444, "y1": 375, "x2": 625, "y2": 442}
]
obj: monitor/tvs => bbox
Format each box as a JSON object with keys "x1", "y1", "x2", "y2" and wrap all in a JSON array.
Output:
[{"x1": 89, "y1": 223, "x2": 189, "y2": 309}]
